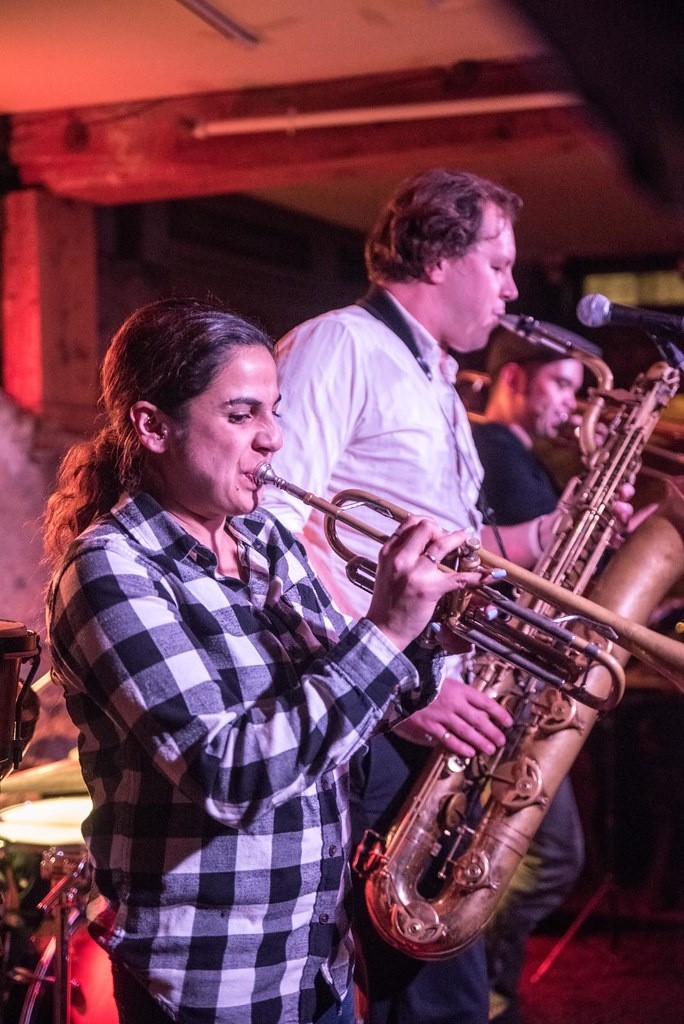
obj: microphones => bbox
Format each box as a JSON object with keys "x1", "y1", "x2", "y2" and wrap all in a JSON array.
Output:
[{"x1": 575, "y1": 292, "x2": 684, "y2": 338}]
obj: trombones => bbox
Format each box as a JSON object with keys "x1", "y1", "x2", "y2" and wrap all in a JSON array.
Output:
[{"x1": 450, "y1": 365, "x2": 684, "y2": 488}]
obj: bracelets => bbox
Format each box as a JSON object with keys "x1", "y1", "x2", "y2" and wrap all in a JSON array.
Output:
[{"x1": 528, "y1": 514, "x2": 549, "y2": 562}]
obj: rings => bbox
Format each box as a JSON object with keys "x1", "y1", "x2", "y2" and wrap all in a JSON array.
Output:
[
  {"x1": 440, "y1": 730, "x2": 452, "y2": 743},
  {"x1": 391, "y1": 527, "x2": 408, "y2": 546},
  {"x1": 420, "y1": 549, "x2": 441, "y2": 568}
]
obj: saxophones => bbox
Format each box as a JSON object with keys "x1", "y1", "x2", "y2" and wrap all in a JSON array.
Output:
[{"x1": 353, "y1": 311, "x2": 683, "y2": 960}]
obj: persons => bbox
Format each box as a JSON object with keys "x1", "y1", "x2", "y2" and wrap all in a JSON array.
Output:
[
  {"x1": 458, "y1": 313, "x2": 597, "y2": 1024},
  {"x1": 252, "y1": 165, "x2": 646, "y2": 1024},
  {"x1": 44, "y1": 279, "x2": 509, "y2": 1024}
]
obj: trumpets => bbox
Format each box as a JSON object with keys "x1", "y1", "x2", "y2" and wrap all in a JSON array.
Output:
[{"x1": 253, "y1": 460, "x2": 683, "y2": 713}]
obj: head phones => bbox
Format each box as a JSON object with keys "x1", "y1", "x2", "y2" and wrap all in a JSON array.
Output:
[{"x1": 359, "y1": 283, "x2": 458, "y2": 384}]
